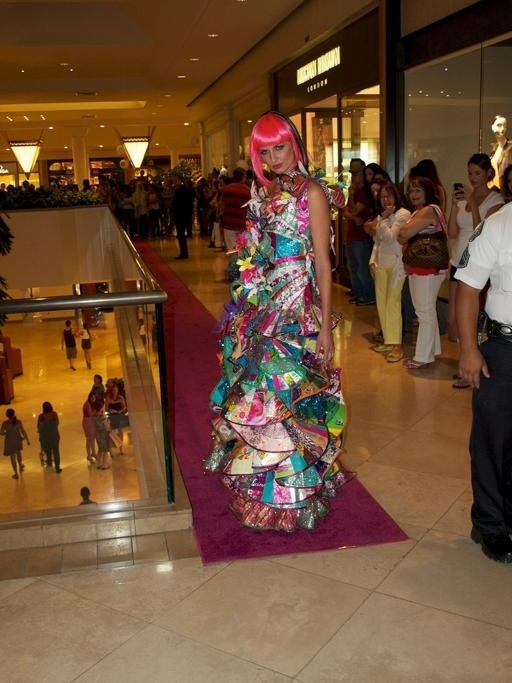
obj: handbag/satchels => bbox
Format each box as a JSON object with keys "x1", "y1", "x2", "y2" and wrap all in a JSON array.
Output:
[{"x1": 401, "y1": 231, "x2": 450, "y2": 270}]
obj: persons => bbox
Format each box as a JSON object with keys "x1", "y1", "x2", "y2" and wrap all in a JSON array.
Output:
[
  {"x1": 500, "y1": 163, "x2": 511, "y2": 204},
  {"x1": 1, "y1": 176, "x2": 105, "y2": 200},
  {"x1": 61, "y1": 320, "x2": 78, "y2": 370},
  {"x1": 0, "y1": 408, "x2": 32, "y2": 478},
  {"x1": 335, "y1": 146, "x2": 497, "y2": 372},
  {"x1": 204, "y1": 114, "x2": 350, "y2": 531},
  {"x1": 137, "y1": 307, "x2": 157, "y2": 363},
  {"x1": 76, "y1": 322, "x2": 94, "y2": 368},
  {"x1": 37, "y1": 401, "x2": 63, "y2": 473},
  {"x1": 451, "y1": 177, "x2": 512, "y2": 561},
  {"x1": 482, "y1": 112, "x2": 510, "y2": 188},
  {"x1": 106, "y1": 160, "x2": 250, "y2": 275},
  {"x1": 79, "y1": 486, "x2": 97, "y2": 504},
  {"x1": 82, "y1": 374, "x2": 130, "y2": 471}
]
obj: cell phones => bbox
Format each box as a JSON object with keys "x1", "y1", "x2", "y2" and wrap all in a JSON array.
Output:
[{"x1": 452, "y1": 182, "x2": 464, "y2": 201}]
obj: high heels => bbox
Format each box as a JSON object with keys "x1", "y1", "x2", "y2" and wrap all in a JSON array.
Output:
[{"x1": 87, "y1": 452, "x2": 111, "y2": 470}]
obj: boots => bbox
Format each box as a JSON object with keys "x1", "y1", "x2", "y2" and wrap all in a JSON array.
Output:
[{"x1": 470, "y1": 525, "x2": 510, "y2": 562}]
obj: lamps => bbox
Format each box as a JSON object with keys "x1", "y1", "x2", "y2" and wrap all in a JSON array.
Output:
[
  {"x1": 115, "y1": 125, "x2": 155, "y2": 171},
  {"x1": 8, "y1": 128, "x2": 46, "y2": 180}
]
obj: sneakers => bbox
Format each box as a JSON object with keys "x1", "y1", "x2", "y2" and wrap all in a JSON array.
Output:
[
  {"x1": 452, "y1": 379, "x2": 471, "y2": 389},
  {"x1": 86, "y1": 362, "x2": 92, "y2": 368},
  {"x1": 348, "y1": 289, "x2": 375, "y2": 307},
  {"x1": 385, "y1": 345, "x2": 405, "y2": 363},
  {"x1": 374, "y1": 341, "x2": 395, "y2": 352},
  {"x1": 12, "y1": 461, "x2": 63, "y2": 479},
  {"x1": 174, "y1": 255, "x2": 189, "y2": 259}
]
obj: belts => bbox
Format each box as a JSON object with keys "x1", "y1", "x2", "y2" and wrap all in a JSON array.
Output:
[{"x1": 489, "y1": 321, "x2": 512, "y2": 341}]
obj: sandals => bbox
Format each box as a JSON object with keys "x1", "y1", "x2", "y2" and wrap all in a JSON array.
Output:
[{"x1": 406, "y1": 356, "x2": 431, "y2": 370}]
